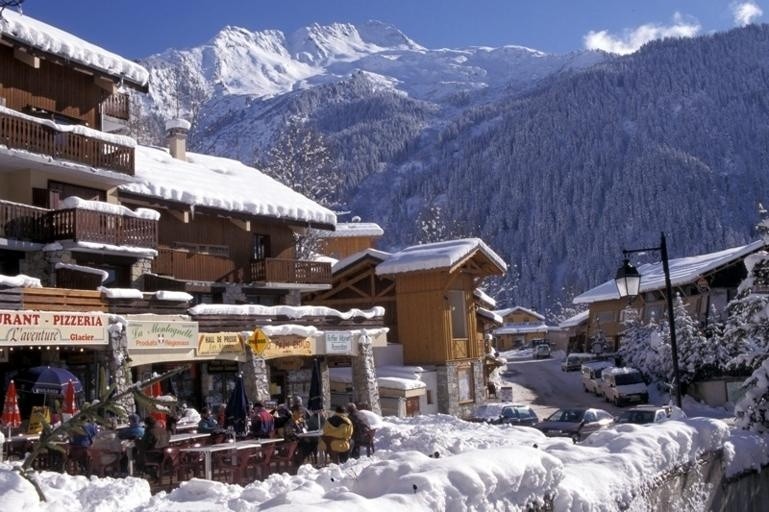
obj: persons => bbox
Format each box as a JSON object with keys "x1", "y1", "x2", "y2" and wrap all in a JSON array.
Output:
[
  {"x1": 291, "y1": 396, "x2": 308, "y2": 420},
  {"x1": 323, "y1": 406, "x2": 355, "y2": 462},
  {"x1": 59, "y1": 413, "x2": 172, "y2": 478},
  {"x1": 250, "y1": 402, "x2": 275, "y2": 434},
  {"x1": 198, "y1": 408, "x2": 219, "y2": 431},
  {"x1": 347, "y1": 403, "x2": 370, "y2": 454},
  {"x1": 288, "y1": 405, "x2": 317, "y2": 464}
]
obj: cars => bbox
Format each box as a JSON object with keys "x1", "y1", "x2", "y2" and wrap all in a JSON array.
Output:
[
  {"x1": 520, "y1": 338, "x2": 552, "y2": 360},
  {"x1": 534, "y1": 406, "x2": 614, "y2": 443},
  {"x1": 560, "y1": 356, "x2": 583, "y2": 373}
]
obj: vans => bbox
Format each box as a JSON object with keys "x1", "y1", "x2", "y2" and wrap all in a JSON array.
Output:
[
  {"x1": 601, "y1": 366, "x2": 649, "y2": 406},
  {"x1": 580, "y1": 361, "x2": 617, "y2": 396}
]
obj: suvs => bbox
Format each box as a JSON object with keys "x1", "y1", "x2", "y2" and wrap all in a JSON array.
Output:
[
  {"x1": 616, "y1": 404, "x2": 689, "y2": 425},
  {"x1": 465, "y1": 403, "x2": 538, "y2": 428}
]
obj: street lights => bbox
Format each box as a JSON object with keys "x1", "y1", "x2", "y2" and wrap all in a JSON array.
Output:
[{"x1": 616, "y1": 232, "x2": 683, "y2": 409}]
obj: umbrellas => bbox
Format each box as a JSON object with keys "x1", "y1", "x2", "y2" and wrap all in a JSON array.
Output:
[{"x1": 8, "y1": 365, "x2": 85, "y2": 409}]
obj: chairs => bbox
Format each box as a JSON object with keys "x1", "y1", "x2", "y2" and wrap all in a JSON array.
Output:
[{"x1": 3, "y1": 408, "x2": 376, "y2": 489}]
